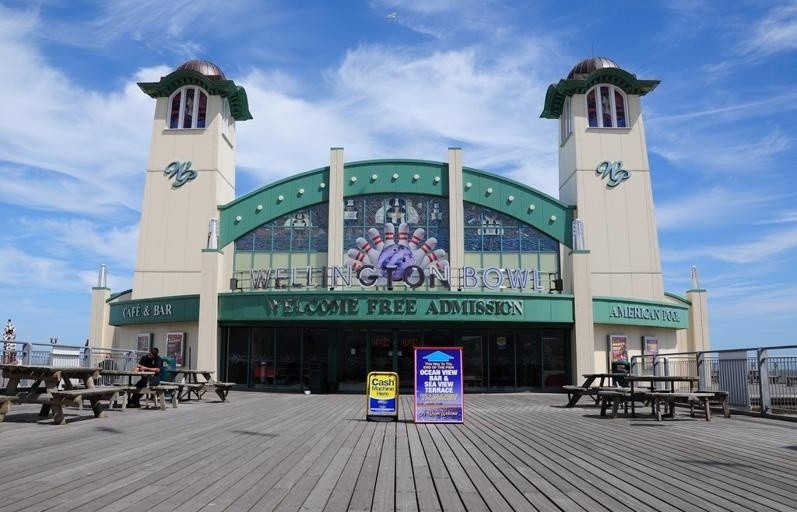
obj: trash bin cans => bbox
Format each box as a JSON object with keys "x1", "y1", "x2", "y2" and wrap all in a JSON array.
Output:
[
  {"x1": 612, "y1": 360, "x2": 631, "y2": 388},
  {"x1": 155, "y1": 355, "x2": 176, "y2": 397}
]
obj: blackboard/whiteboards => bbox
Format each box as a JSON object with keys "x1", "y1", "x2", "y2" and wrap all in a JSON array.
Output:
[{"x1": 413, "y1": 346, "x2": 463, "y2": 422}]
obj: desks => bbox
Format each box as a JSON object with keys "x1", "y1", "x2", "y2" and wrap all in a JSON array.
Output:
[
  {"x1": 174, "y1": 369, "x2": 225, "y2": 403},
  {"x1": 0, "y1": 361, "x2": 103, "y2": 428},
  {"x1": 584, "y1": 372, "x2": 702, "y2": 423},
  {"x1": 102, "y1": 369, "x2": 156, "y2": 407}
]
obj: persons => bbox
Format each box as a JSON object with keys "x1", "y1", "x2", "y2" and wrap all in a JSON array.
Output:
[
  {"x1": 95, "y1": 353, "x2": 117, "y2": 385},
  {"x1": 613, "y1": 351, "x2": 632, "y2": 388},
  {"x1": 127, "y1": 347, "x2": 163, "y2": 407}
]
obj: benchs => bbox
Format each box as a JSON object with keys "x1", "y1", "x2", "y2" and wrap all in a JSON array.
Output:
[
  {"x1": 214, "y1": 382, "x2": 237, "y2": 403},
  {"x1": 48, "y1": 383, "x2": 137, "y2": 420},
  {"x1": 561, "y1": 385, "x2": 586, "y2": 407},
  {"x1": 644, "y1": 389, "x2": 731, "y2": 422},
  {"x1": 147, "y1": 379, "x2": 202, "y2": 410}
]
